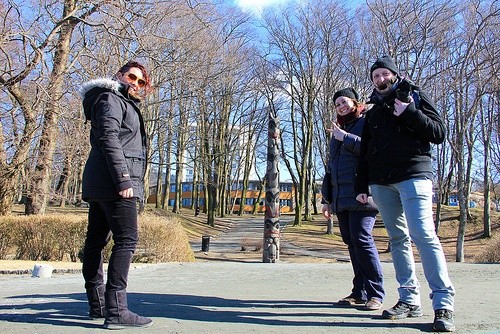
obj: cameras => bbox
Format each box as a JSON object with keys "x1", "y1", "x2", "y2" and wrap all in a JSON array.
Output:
[{"x1": 396, "y1": 89, "x2": 410, "y2": 103}]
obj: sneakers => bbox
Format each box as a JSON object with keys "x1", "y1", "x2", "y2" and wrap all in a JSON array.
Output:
[
  {"x1": 433, "y1": 308, "x2": 455, "y2": 332},
  {"x1": 363, "y1": 297, "x2": 384, "y2": 310},
  {"x1": 338, "y1": 292, "x2": 366, "y2": 305},
  {"x1": 382, "y1": 300, "x2": 423, "y2": 320}
]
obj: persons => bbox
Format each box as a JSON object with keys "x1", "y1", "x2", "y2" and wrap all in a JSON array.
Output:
[
  {"x1": 355, "y1": 54, "x2": 456, "y2": 332},
  {"x1": 78, "y1": 60, "x2": 153, "y2": 329},
  {"x1": 320, "y1": 87, "x2": 385, "y2": 310}
]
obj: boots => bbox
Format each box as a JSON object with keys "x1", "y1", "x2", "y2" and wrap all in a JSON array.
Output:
[
  {"x1": 86, "y1": 284, "x2": 107, "y2": 322},
  {"x1": 103, "y1": 290, "x2": 154, "y2": 330}
]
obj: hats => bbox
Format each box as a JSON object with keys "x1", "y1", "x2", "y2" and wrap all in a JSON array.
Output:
[
  {"x1": 332, "y1": 86, "x2": 359, "y2": 108},
  {"x1": 370, "y1": 54, "x2": 402, "y2": 83}
]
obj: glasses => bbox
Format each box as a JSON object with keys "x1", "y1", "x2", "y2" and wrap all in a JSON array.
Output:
[{"x1": 123, "y1": 72, "x2": 146, "y2": 87}]
